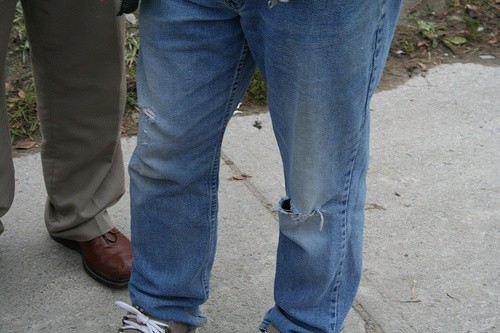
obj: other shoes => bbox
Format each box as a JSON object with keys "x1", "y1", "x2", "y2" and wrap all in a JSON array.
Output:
[{"x1": 114, "y1": 299, "x2": 197, "y2": 332}]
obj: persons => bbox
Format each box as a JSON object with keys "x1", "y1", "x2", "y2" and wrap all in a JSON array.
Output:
[
  {"x1": 113, "y1": 0, "x2": 404, "y2": 333},
  {"x1": 1, "y1": 0, "x2": 132, "y2": 287}
]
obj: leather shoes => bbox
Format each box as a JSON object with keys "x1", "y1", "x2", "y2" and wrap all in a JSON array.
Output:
[{"x1": 51, "y1": 225, "x2": 132, "y2": 287}]
